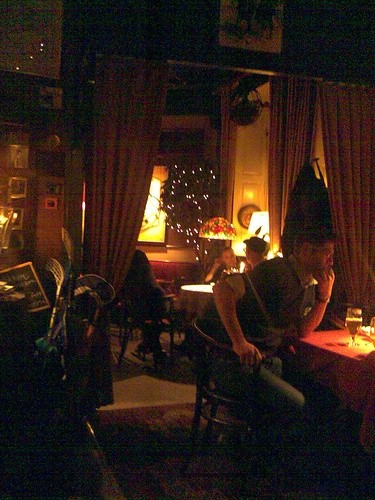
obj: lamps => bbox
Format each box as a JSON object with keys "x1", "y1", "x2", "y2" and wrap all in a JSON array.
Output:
[
  {"x1": 248, "y1": 211, "x2": 270, "y2": 241},
  {"x1": 197, "y1": 215, "x2": 237, "y2": 241}
]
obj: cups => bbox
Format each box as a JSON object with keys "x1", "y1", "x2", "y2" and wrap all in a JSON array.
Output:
[{"x1": 370, "y1": 316, "x2": 375, "y2": 341}]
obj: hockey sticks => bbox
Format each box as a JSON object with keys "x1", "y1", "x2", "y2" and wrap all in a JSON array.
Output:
[{"x1": 44, "y1": 227, "x2": 116, "y2": 355}]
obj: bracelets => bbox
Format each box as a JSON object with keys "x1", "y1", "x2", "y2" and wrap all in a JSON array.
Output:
[{"x1": 317, "y1": 294, "x2": 330, "y2": 302}]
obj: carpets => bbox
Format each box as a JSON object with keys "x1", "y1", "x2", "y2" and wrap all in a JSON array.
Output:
[{"x1": 92, "y1": 402, "x2": 330, "y2": 500}]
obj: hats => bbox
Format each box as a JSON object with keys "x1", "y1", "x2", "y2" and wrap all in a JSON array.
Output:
[{"x1": 243, "y1": 237, "x2": 268, "y2": 252}]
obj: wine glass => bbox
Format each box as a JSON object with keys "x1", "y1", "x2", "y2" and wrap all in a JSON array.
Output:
[{"x1": 345, "y1": 308, "x2": 362, "y2": 347}]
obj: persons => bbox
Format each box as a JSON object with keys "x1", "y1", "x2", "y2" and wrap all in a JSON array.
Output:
[
  {"x1": 126, "y1": 251, "x2": 171, "y2": 369},
  {"x1": 210, "y1": 224, "x2": 336, "y2": 500},
  {"x1": 204, "y1": 237, "x2": 270, "y2": 282}
]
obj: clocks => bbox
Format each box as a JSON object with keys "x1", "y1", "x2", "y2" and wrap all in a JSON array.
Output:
[{"x1": 238, "y1": 204, "x2": 261, "y2": 228}]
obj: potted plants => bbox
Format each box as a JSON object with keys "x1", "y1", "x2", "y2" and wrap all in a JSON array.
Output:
[{"x1": 225, "y1": 86, "x2": 263, "y2": 127}]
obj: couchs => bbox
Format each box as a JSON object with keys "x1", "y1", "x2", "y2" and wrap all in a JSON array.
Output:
[{"x1": 116, "y1": 260, "x2": 198, "y2": 312}]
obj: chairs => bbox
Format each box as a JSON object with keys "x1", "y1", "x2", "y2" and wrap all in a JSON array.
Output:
[{"x1": 67, "y1": 265, "x2": 345, "y2": 470}]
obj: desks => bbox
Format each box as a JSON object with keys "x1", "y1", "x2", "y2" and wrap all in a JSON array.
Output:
[
  {"x1": 295, "y1": 328, "x2": 375, "y2": 413},
  {"x1": 180, "y1": 284, "x2": 214, "y2": 319}
]
